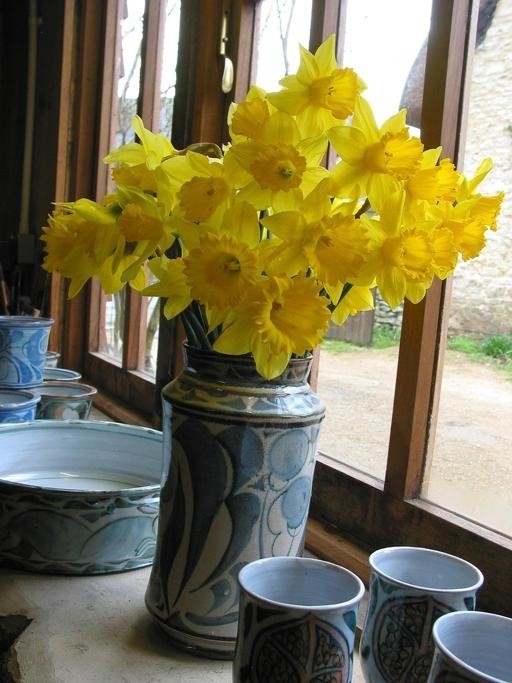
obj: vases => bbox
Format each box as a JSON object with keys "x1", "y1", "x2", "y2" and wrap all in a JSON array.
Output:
[{"x1": 144, "y1": 338, "x2": 326, "y2": 663}]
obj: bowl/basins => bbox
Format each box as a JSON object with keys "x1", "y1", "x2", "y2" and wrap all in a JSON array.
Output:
[{"x1": 0, "y1": 418, "x2": 163, "y2": 576}]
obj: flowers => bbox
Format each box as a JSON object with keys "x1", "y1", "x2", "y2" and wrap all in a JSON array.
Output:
[{"x1": 39, "y1": 33, "x2": 504, "y2": 383}]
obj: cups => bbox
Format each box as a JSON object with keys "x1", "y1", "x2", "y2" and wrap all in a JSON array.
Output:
[
  {"x1": 0, "y1": 315, "x2": 98, "y2": 424},
  {"x1": 232, "y1": 546, "x2": 512, "y2": 682}
]
